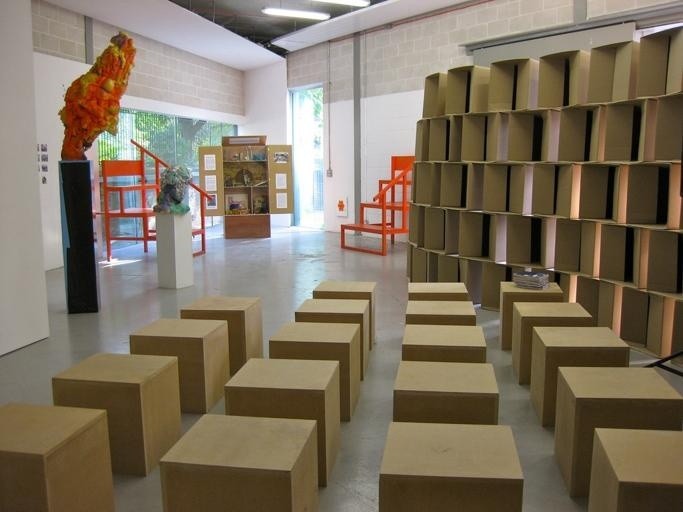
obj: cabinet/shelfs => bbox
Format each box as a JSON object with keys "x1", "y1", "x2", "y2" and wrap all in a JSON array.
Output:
[
  {"x1": 407, "y1": 24, "x2": 683, "y2": 362},
  {"x1": 198, "y1": 135, "x2": 294, "y2": 240}
]
